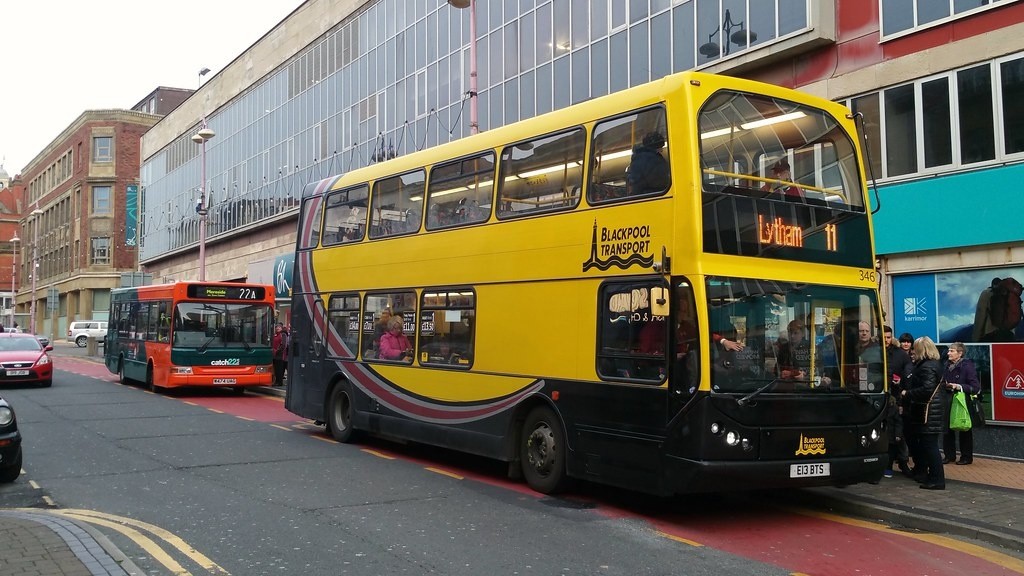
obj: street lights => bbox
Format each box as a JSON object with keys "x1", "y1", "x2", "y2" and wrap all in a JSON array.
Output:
[
  {"x1": 192, "y1": 113, "x2": 215, "y2": 283},
  {"x1": 9, "y1": 230, "x2": 20, "y2": 327},
  {"x1": 28, "y1": 200, "x2": 45, "y2": 336},
  {"x1": 447, "y1": 0, "x2": 479, "y2": 137},
  {"x1": 699, "y1": 9, "x2": 757, "y2": 60}
]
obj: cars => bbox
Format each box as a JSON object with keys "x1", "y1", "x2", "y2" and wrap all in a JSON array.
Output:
[
  {"x1": 3, "y1": 327, "x2": 17, "y2": 333},
  {"x1": 0, "y1": 332, "x2": 53, "y2": 387},
  {"x1": 0, "y1": 395, "x2": 23, "y2": 484},
  {"x1": 35, "y1": 334, "x2": 49, "y2": 348}
]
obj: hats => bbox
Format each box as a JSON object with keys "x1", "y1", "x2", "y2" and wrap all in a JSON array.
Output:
[
  {"x1": 771, "y1": 159, "x2": 790, "y2": 171},
  {"x1": 642, "y1": 132, "x2": 665, "y2": 148}
]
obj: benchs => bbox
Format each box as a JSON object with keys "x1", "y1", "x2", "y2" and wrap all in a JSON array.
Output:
[
  {"x1": 572, "y1": 183, "x2": 627, "y2": 205},
  {"x1": 373, "y1": 337, "x2": 381, "y2": 359},
  {"x1": 323, "y1": 197, "x2": 515, "y2": 244}
]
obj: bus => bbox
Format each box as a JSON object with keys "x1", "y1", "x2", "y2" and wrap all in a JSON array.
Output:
[
  {"x1": 285, "y1": 71, "x2": 891, "y2": 503},
  {"x1": 105, "y1": 281, "x2": 281, "y2": 394}
]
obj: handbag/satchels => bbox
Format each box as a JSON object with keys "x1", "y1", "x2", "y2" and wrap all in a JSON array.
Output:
[
  {"x1": 911, "y1": 403, "x2": 928, "y2": 424},
  {"x1": 969, "y1": 386, "x2": 985, "y2": 429},
  {"x1": 950, "y1": 385, "x2": 972, "y2": 431}
]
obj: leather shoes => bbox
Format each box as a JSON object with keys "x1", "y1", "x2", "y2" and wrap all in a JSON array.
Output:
[
  {"x1": 942, "y1": 456, "x2": 956, "y2": 464},
  {"x1": 957, "y1": 457, "x2": 973, "y2": 465},
  {"x1": 919, "y1": 482, "x2": 945, "y2": 490},
  {"x1": 918, "y1": 478, "x2": 931, "y2": 483}
]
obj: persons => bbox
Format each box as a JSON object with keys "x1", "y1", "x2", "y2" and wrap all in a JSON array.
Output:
[
  {"x1": 630, "y1": 132, "x2": 669, "y2": 195},
  {"x1": 13, "y1": 320, "x2": 19, "y2": 328},
  {"x1": 943, "y1": 342, "x2": 979, "y2": 465},
  {"x1": 860, "y1": 320, "x2": 914, "y2": 478},
  {"x1": 373, "y1": 312, "x2": 391, "y2": 359},
  {"x1": 972, "y1": 278, "x2": 1015, "y2": 342},
  {"x1": 760, "y1": 161, "x2": 805, "y2": 197},
  {"x1": 0, "y1": 321, "x2": 4, "y2": 333},
  {"x1": 774, "y1": 320, "x2": 841, "y2": 380},
  {"x1": 273, "y1": 322, "x2": 290, "y2": 385},
  {"x1": 901, "y1": 336, "x2": 947, "y2": 490},
  {"x1": 379, "y1": 315, "x2": 412, "y2": 359},
  {"x1": 433, "y1": 338, "x2": 458, "y2": 365},
  {"x1": 639, "y1": 296, "x2": 743, "y2": 367}
]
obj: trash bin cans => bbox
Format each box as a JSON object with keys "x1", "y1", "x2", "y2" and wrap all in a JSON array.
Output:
[
  {"x1": 104, "y1": 335, "x2": 108, "y2": 356},
  {"x1": 86, "y1": 336, "x2": 96, "y2": 357}
]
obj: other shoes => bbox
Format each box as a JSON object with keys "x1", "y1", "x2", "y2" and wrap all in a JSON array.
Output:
[
  {"x1": 898, "y1": 463, "x2": 910, "y2": 471},
  {"x1": 273, "y1": 382, "x2": 283, "y2": 386},
  {"x1": 906, "y1": 467, "x2": 920, "y2": 478},
  {"x1": 884, "y1": 469, "x2": 894, "y2": 478}
]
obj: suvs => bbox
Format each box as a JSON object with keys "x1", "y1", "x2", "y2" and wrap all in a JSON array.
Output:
[{"x1": 67, "y1": 319, "x2": 109, "y2": 348}]
